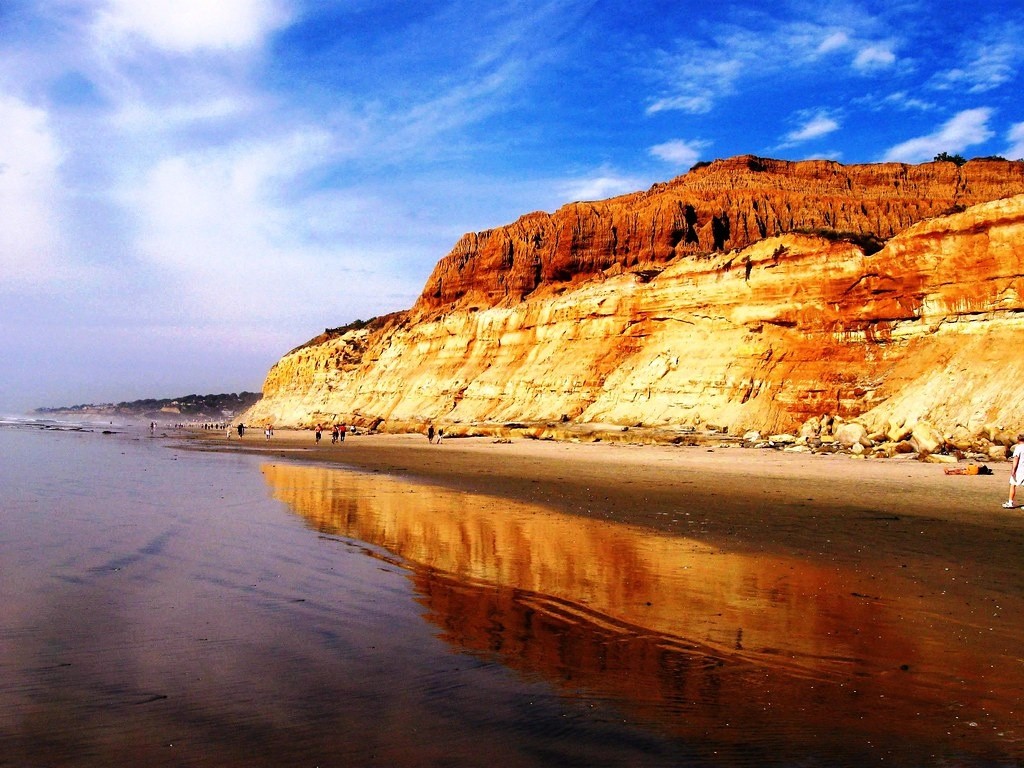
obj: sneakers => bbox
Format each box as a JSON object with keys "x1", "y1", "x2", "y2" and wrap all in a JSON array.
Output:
[{"x1": 1002, "y1": 502, "x2": 1014, "y2": 508}]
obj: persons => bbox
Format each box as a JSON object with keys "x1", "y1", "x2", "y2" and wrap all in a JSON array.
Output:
[
  {"x1": 315, "y1": 423, "x2": 371, "y2": 444},
  {"x1": 1002, "y1": 434, "x2": 1024, "y2": 510},
  {"x1": 428, "y1": 425, "x2": 444, "y2": 444},
  {"x1": 944, "y1": 462, "x2": 992, "y2": 475},
  {"x1": 149, "y1": 421, "x2": 274, "y2": 442}
]
